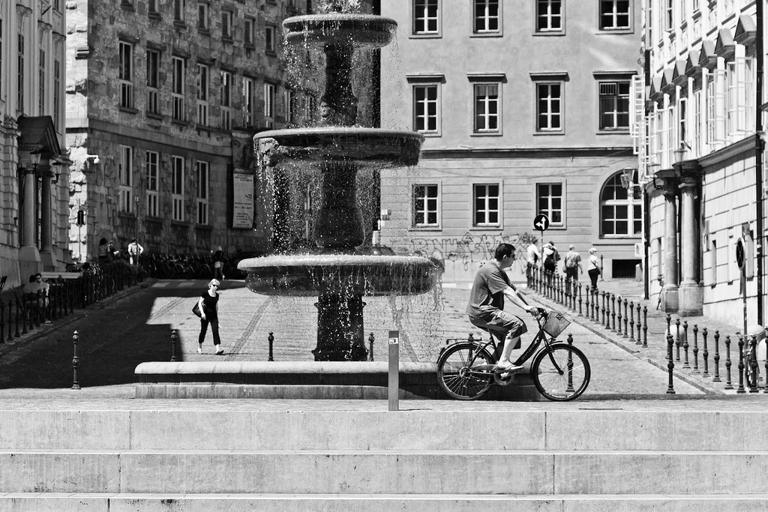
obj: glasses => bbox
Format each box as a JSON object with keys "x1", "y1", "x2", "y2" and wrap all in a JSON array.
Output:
[{"x1": 510, "y1": 254, "x2": 516, "y2": 258}]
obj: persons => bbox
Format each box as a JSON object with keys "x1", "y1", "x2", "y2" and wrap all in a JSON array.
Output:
[
  {"x1": 527, "y1": 237, "x2": 602, "y2": 295},
  {"x1": 24, "y1": 272, "x2": 50, "y2": 309},
  {"x1": 98, "y1": 238, "x2": 121, "y2": 263},
  {"x1": 198, "y1": 278, "x2": 224, "y2": 355},
  {"x1": 214, "y1": 246, "x2": 227, "y2": 280},
  {"x1": 128, "y1": 238, "x2": 145, "y2": 265},
  {"x1": 465, "y1": 243, "x2": 540, "y2": 372}
]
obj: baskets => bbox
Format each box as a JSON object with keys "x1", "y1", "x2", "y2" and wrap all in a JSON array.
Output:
[{"x1": 542, "y1": 311, "x2": 571, "y2": 338}]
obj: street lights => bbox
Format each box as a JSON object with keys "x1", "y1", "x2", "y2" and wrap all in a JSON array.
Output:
[{"x1": 134, "y1": 195, "x2": 139, "y2": 265}]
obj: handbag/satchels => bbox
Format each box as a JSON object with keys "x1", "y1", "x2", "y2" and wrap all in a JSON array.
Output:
[
  {"x1": 596, "y1": 267, "x2": 601, "y2": 274},
  {"x1": 192, "y1": 301, "x2": 207, "y2": 317}
]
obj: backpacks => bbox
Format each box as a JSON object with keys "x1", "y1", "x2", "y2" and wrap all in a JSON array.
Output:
[{"x1": 567, "y1": 253, "x2": 576, "y2": 268}]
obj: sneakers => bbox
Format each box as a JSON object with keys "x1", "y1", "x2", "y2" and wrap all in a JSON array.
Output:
[
  {"x1": 496, "y1": 360, "x2": 523, "y2": 373},
  {"x1": 198, "y1": 347, "x2": 203, "y2": 354},
  {"x1": 216, "y1": 349, "x2": 224, "y2": 355}
]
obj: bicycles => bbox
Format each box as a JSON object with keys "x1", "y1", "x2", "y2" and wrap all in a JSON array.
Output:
[
  {"x1": 121, "y1": 251, "x2": 232, "y2": 276},
  {"x1": 437, "y1": 306, "x2": 590, "y2": 401}
]
